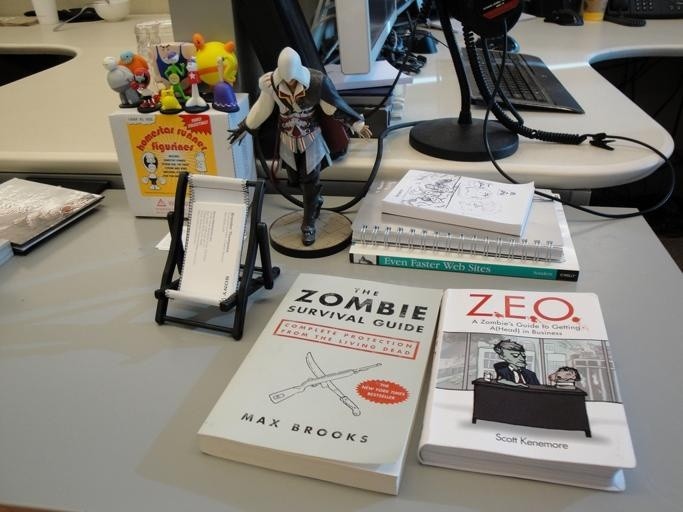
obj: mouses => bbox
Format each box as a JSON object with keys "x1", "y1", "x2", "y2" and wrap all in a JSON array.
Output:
[
  {"x1": 543, "y1": 6, "x2": 584, "y2": 26},
  {"x1": 476, "y1": 34, "x2": 519, "y2": 51}
]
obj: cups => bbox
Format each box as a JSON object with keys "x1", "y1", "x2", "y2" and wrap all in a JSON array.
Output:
[{"x1": 132, "y1": 21, "x2": 174, "y2": 52}]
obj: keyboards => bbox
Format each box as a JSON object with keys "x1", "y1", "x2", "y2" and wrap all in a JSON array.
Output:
[{"x1": 460, "y1": 45, "x2": 585, "y2": 114}]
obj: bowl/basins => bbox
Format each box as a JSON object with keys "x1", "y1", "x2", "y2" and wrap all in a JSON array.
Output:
[{"x1": 94, "y1": 0, "x2": 131, "y2": 23}]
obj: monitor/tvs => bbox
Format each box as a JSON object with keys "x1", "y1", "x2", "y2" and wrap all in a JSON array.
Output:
[{"x1": 333, "y1": 0, "x2": 414, "y2": 75}]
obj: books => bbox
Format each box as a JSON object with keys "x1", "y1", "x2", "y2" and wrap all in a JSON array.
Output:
[
  {"x1": 198, "y1": 274, "x2": 443, "y2": 495},
  {"x1": 417, "y1": 288, "x2": 636, "y2": 491},
  {"x1": 349, "y1": 170, "x2": 580, "y2": 282},
  {"x1": 0, "y1": 177, "x2": 104, "y2": 266}
]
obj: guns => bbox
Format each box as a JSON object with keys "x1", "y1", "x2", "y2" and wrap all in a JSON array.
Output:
[{"x1": 270, "y1": 364, "x2": 381, "y2": 404}]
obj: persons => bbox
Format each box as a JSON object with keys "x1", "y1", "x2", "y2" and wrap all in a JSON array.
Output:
[{"x1": 225, "y1": 46, "x2": 375, "y2": 245}]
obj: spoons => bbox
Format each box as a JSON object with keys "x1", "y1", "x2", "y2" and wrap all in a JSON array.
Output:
[{"x1": 52, "y1": 4, "x2": 105, "y2": 32}]
